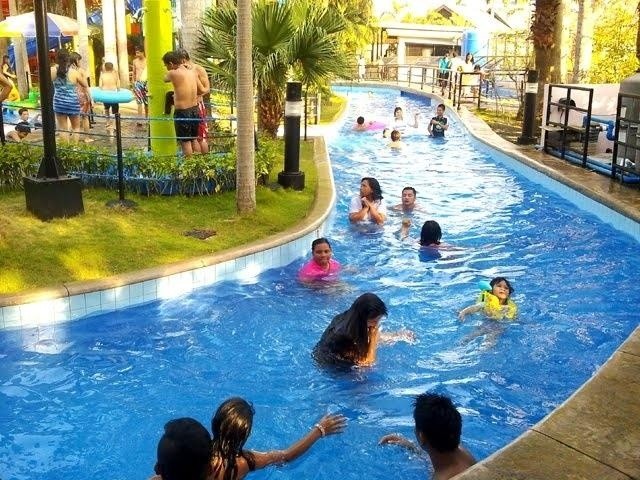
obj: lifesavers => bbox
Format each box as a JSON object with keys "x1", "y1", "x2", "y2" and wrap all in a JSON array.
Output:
[{"x1": 89, "y1": 87, "x2": 134, "y2": 103}]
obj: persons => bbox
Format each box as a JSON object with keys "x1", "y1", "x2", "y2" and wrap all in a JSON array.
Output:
[
  {"x1": 393, "y1": 187, "x2": 425, "y2": 210},
  {"x1": 394, "y1": 108, "x2": 403, "y2": 120},
  {"x1": 154, "y1": 417, "x2": 211, "y2": 480},
  {"x1": 437, "y1": 50, "x2": 481, "y2": 104},
  {"x1": 428, "y1": 104, "x2": 449, "y2": 137},
  {"x1": 353, "y1": 116, "x2": 367, "y2": 131},
  {"x1": 2, "y1": 49, "x2": 212, "y2": 158},
  {"x1": 298, "y1": 237, "x2": 340, "y2": 276},
  {"x1": 389, "y1": 131, "x2": 407, "y2": 148},
  {"x1": 382, "y1": 128, "x2": 390, "y2": 138},
  {"x1": 312, "y1": 292, "x2": 387, "y2": 366},
  {"x1": 459, "y1": 276, "x2": 518, "y2": 323},
  {"x1": 401, "y1": 219, "x2": 443, "y2": 249},
  {"x1": 209, "y1": 396, "x2": 348, "y2": 480},
  {"x1": 348, "y1": 177, "x2": 386, "y2": 225},
  {"x1": 377, "y1": 392, "x2": 477, "y2": 480}
]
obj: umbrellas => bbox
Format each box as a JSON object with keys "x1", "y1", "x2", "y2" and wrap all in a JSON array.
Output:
[{"x1": 0, "y1": 11, "x2": 92, "y2": 38}]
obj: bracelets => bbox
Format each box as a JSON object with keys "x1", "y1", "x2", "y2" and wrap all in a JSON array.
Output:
[{"x1": 314, "y1": 423, "x2": 326, "y2": 437}]
